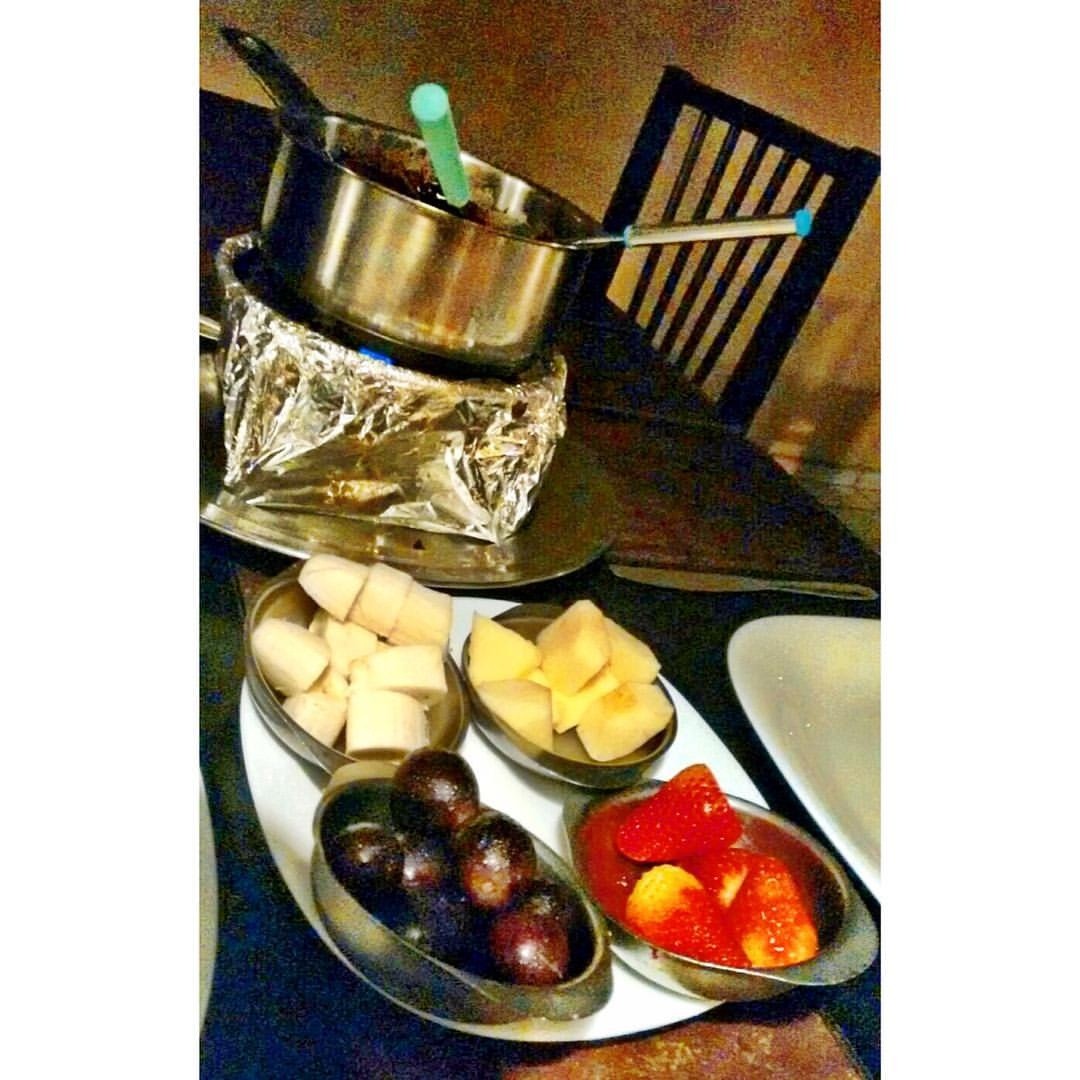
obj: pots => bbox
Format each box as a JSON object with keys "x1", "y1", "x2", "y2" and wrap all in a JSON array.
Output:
[{"x1": 218, "y1": 25, "x2": 601, "y2": 373}]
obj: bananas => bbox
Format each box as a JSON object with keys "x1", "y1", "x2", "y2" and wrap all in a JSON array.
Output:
[{"x1": 256, "y1": 555, "x2": 457, "y2": 760}]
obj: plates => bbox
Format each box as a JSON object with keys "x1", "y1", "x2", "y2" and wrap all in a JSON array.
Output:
[
  {"x1": 728, "y1": 615, "x2": 881, "y2": 906},
  {"x1": 240, "y1": 596, "x2": 774, "y2": 1047}
]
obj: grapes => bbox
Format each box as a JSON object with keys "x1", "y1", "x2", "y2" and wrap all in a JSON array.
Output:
[{"x1": 331, "y1": 745, "x2": 591, "y2": 988}]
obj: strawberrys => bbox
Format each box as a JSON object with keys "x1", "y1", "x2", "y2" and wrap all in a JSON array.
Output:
[{"x1": 615, "y1": 760, "x2": 821, "y2": 973}]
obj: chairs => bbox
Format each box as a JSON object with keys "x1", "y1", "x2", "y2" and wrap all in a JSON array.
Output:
[{"x1": 590, "y1": 65, "x2": 880, "y2": 437}]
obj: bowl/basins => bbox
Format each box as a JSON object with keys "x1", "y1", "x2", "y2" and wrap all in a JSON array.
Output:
[
  {"x1": 312, "y1": 759, "x2": 615, "y2": 1025},
  {"x1": 561, "y1": 778, "x2": 879, "y2": 1000},
  {"x1": 462, "y1": 603, "x2": 678, "y2": 789},
  {"x1": 244, "y1": 571, "x2": 471, "y2": 774}
]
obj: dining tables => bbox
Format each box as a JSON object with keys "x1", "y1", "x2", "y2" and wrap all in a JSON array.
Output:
[{"x1": 200, "y1": 88, "x2": 881, "y2": 1080}]
